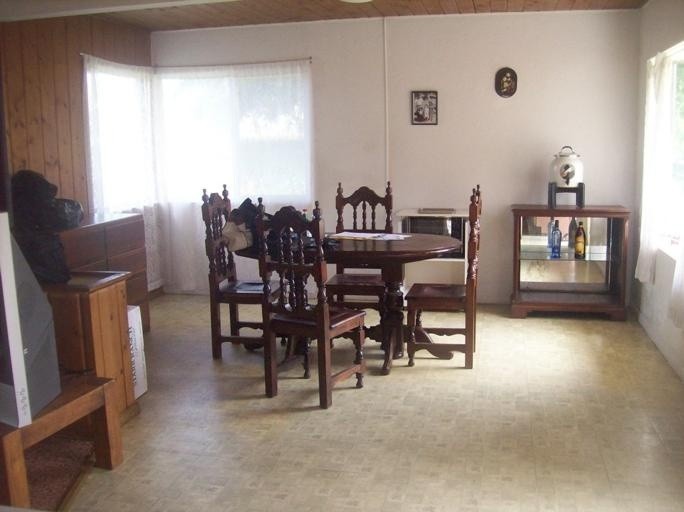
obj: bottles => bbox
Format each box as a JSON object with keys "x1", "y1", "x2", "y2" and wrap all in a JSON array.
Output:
[
  {"x1": 547, "y1": 217, "x2": 555, "y2": 248},
  {"x1": 550, "y1": 221, "x2": 561, "y2": 256},
  {"x1": 575, "y1": 221, "x2": 586, "y2": 259},
  {"x1": 567, "y1": 217, "x2": 577, "y2": 249},
  {"x1": 301, "y1": 209, "x2": 308, "y2": 222}
]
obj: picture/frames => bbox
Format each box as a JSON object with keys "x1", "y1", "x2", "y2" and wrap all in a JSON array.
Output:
[{"x1": 412, "y1": 91, "x2": 437, "y2": 125}]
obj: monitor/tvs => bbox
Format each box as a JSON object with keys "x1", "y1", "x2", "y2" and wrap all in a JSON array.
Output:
[{"x1": 0, "y1": 212, "x2": 62, "y2": 429}]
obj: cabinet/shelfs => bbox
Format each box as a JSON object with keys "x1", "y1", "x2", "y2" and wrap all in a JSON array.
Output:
[
  {"x1": 511, "y1": 205, "x2": 632, "y2": 321},
  {"x1": 56, "y1": 213, "x2": 150, "y2": 332},
  {"x1": 43, "y1": 271, "x2": 141, "y2": 437}
]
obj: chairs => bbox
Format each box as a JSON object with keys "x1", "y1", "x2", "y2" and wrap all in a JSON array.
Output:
[
  {"x1": 202, "y1": 184, "x2": 284, "y2": 359},
  {"x1": 405, "y1": 185, "x2": 482, "y2": 368},
  {"x1": 325, "y1": 182, "x2": 393, "y2": 349},
  {"x1": 255, "y1": 197, "x2": 366, "y2": 409}
]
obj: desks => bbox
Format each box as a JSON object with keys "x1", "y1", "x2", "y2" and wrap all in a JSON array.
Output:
[{"x1": 0, "y1": 375, "x2": 123, "y2": 512}]
